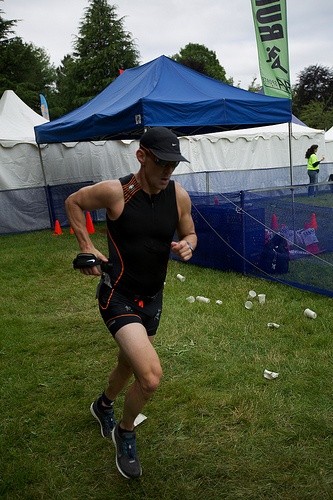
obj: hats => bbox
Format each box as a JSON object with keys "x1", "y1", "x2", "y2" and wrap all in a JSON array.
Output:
[{"x1": 140, "y1": 127, "x2": 190, "y2": 164}]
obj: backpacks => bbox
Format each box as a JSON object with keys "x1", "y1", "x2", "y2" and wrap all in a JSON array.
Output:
[{"x1": 264, "y1": 234, "x2": 289, "y2": 273}]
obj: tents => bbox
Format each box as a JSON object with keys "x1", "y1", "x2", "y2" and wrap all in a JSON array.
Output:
[
  {"x1": 0, "y1": 89, "x2": 331, "y2": 235},
  {"x1": 34, "y1": 55, "x2": 298, "y2": 251},
  {"x1": 325, "y1": 125, "x2": 333, "y2": 192}
]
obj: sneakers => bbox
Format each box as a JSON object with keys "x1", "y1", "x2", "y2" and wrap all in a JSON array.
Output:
[
  {"x1": 90, "y1": 397, "x2": 117, "y2": 443},
  {"x1": 112, "y1": 421, "x2": 142, "y2": 478}
]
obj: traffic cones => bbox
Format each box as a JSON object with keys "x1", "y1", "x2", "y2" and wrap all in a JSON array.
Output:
[
  {"x1": 52, "y1": 219, "x2": 63, "y2": 236},
  {"x1": 271, "y1": 213, "x2": 279, "y2": 230},
  {"x1": 85, "y1": 211, "x2": 95, "y2": 235},
  {"x1": 305, "y1": 213, "x2": 318, "y2": 230}
]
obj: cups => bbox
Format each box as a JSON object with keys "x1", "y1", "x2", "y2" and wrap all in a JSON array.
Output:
[
  {"x1": 186, "y1": 296, "x2": 195, "y2": 303},
  {"x1": 303, "y1": 308, "x2": 317, "y2": 319},
  {"x1": 177, "y1": 274, "x2": 186, "y2": 282},
  {"x1": 245, "y1": 301, "x2": 253, "y2": 310},
  {"x1": 258, "y1": 294, "x2": 266, "y2": 303},
  {"x1": 246, "y1": 290, "x2": 256, "y2": 301},
  {"x1": 196, "y1": 296, "x2": 210, "y2": 303}
]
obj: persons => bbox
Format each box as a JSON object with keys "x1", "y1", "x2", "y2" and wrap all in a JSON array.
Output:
[
  {"x1": 298, "y1": 144, "x2": 325, "y2": 200},
  {"x1": 65, "y1": 127, "x2": 197, "y2": 479}
]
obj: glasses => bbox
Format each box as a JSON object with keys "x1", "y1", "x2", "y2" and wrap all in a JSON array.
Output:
[{"x1": 148, "y1": 151, "x2": 180, "y2": 167}]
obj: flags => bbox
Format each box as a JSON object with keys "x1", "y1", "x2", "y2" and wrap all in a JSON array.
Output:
[
  {"x1": 120, "y1": 70, "x2": 123, "y2": 74},
  {"x1": 39, "y1": 93, "x2": 49, "y2": 120},
  {"x1": 251, "y1": 0, "x2": 292, "y2": 100}
]
